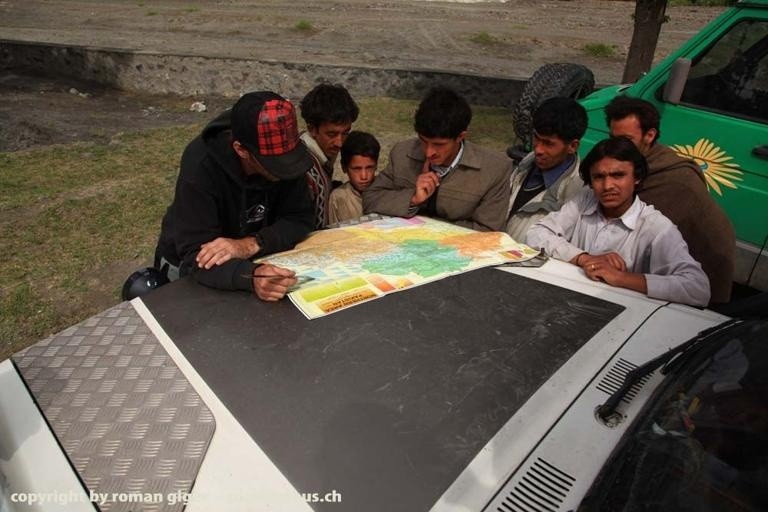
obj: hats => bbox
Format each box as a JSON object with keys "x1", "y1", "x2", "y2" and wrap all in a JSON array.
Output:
[{"x1": 230, "y1": 91, "x2": 314, "y2": 180}]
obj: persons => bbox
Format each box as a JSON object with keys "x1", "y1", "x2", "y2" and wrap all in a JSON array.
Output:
[
  {"x1": 503, "y1": 96, "x2": 592, "y2": 244},
  {"x1": 525, "y1": 134, "x2": 712, "y2": 306},
  {"x1": 154, "y1": 91, "x2": 317, "y2": 303},
  {"x1": 327, "y1": 130, "x2": 382, "y2": 225},
  {"x1": 292, "y1": 83, "x2": 358, "y2": 230},
  {"x1": 362, "y1": 82, "x2": 515, "y2": 232},
  {"x1": 599, "y1": 94, "x2": 738, "y2": 309}
]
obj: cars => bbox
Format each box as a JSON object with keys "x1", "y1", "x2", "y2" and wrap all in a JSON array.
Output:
[{"x1": 0, "y1": 214, "x2": 767, "y2": 512}]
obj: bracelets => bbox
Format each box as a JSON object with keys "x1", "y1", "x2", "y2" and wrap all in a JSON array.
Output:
[{"x1": 576, "y1": 251, "x2": 589, "y2": 266}]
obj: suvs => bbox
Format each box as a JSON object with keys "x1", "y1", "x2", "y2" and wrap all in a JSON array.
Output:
[{"x1": 513, "y1": 0, "x2": 767, "y2": 291}]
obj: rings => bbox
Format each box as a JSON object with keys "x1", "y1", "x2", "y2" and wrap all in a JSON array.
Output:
[{"x1": 591, "y1": 264, "x2": 595, "y2": 270}]
